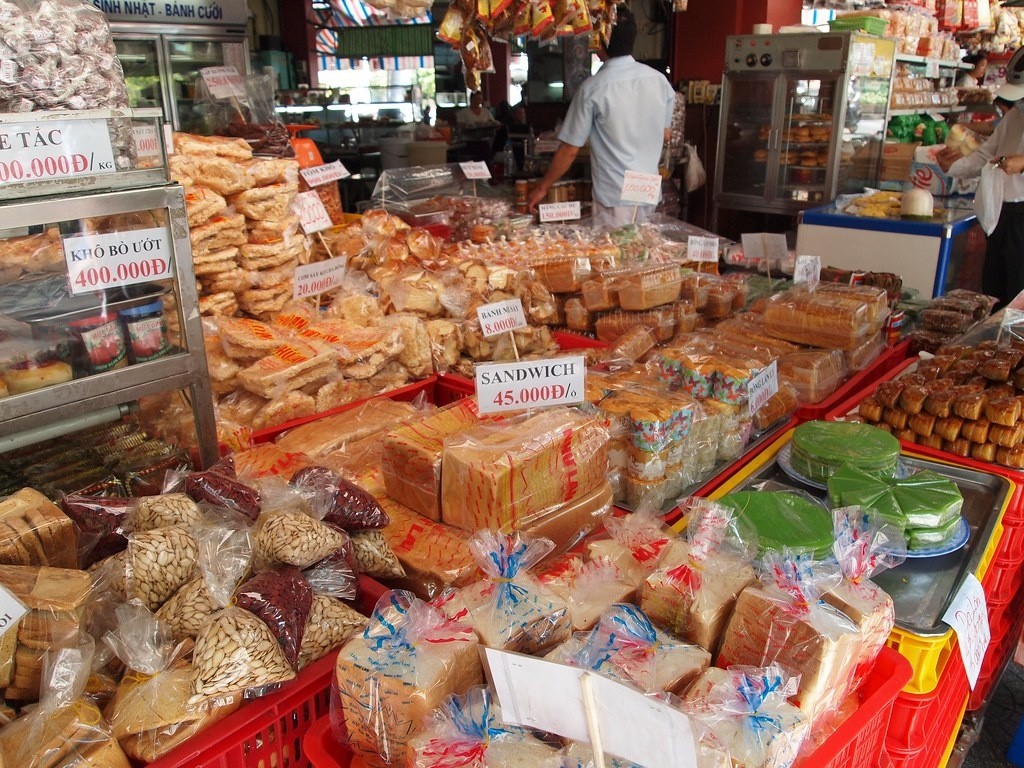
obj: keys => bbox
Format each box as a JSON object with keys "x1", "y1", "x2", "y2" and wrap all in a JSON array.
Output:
[{"x1": 992, "y1": 156, "x2": 1005, "y2": 169}]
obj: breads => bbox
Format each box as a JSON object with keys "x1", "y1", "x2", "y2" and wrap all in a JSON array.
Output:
[{"x1": 0, "y1": 0, "x2": 1023, "y2": 768}]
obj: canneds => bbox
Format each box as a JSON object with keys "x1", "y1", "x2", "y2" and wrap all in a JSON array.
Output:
[
  {"x1": 515, "y1": 180, "x2": 528, "y2": 202},
  {"x1": 528, "y1": 179, "x2": 539, "y2": 192},
  {"x1": 560, "y1": 181, "x2": 568, "y2": 201},
  {"x1": 568, "y1": 180, "x2": 577, "y2": 200},
  {"x1": 547, "y1": 183, "x2": 561, "y2": 202},
  {"x1": 119, "y1": 302, "x2": 171, "y2": 362},
  {"x1": 583, "y1": 180, "x2": 592, "y2": 200},
  {"x1": 516, "y1": 202, "x2": 528, "y2": 214},
  {"x1": 576, "y1": 179, "x2": 584, "y2": 200},
  {"x1": 67, "y1": 311, "x2": 128, "y2": 375}
]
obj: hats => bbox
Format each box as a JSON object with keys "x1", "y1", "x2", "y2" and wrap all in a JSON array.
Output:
[{"x1": 993, "y1": 45, "x2": 1024, "y2": 101}]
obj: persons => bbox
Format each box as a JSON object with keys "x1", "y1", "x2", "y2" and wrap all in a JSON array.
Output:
[
  {"x1": 423, "y1": 105, "x2": 432, "y2": 126},
  {"x1": 527, "y1": 7, "x2": 677, "y2": 226},
  {"x1": 956, "y1": 53, "x2": 987, "y2": 87},
  {"x1": 935, "y1": 45, "x2": 1024, "y2": 314},
  {"x1": 456, "y1": 91, "x2": 500, "y2": 160}
]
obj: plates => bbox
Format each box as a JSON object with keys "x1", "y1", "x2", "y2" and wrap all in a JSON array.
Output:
[
  {"x1": 778, "y1": 441, "x2": 908, "y2": 490},
  {"x1": 824, "y1": 495, "x2": 970, "y2": 558}
]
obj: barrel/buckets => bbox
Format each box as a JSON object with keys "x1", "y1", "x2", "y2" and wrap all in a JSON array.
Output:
[
  {"x1": 378, "y1": 137, "x2": 411, "y2": 170},
  {"x1": 407, "y1": 140, "x2": 448, "y2": 168}
]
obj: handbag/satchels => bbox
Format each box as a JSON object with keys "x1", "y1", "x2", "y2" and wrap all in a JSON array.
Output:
[
  {"x1": 971, "y1": 158, "x2": 1006, "y2": 236},
  {"x1": 684, "y1": 143, "x2": 706, "y2": 192}
]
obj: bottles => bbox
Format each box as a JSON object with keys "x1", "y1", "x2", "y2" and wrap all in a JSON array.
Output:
[{"x1": 504, "y1": 140, "x2": 516, "y2": 177}]
